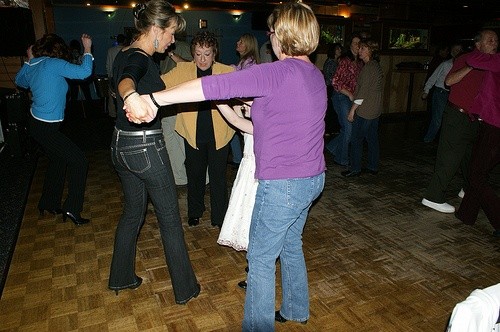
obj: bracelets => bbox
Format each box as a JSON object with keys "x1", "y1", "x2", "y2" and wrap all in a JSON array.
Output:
[
  {"x1": 123, "y1": 91, "x2": 136, "y2": 102},
  {"x1": 169, "y1": 52, "x2": 176, "y2": 58},
  {"x1": 150, "y1": 93, "x2": 161, "y2": 110}
]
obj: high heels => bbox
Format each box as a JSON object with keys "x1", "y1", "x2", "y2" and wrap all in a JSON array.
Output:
[
  {"x1": 178, "y1": 283, "x2": 201, "y2": 305},
  {"x1": 62, "y1": 209, "x2": 89, "y2": 225},
  {"x1": 39, "y1": 208, "x2": 65, "y2": 216},
  {"x1": 114, "y1": 276, "x2": 142, "y2": 296},
  {"x1": 275, "y1": 310, "x2": 307, "y2": 325}
]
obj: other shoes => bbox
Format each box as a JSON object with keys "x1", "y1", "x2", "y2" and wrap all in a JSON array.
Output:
[
  {"x1": 458, "y1": 187, "x2": 466, "y2": 199},
  {"x1": 340, "y1": 170, "x2": 360, "y2": 177},
  {"x1": 211, "y1": 222, "x2": 222, "y2": 230},
  {"x1": 238, "y1": 280, "x2": 248, "y2": 289},
  {"x1": 188, "y1": 217, "x2": 199, "y2": 227},
  {"x1": 421, "y1": 197, "x2": 455, "y2": 213}
]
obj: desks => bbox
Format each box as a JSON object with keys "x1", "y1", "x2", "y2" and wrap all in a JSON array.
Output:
[{"x1": 391, "y1": 69, "x2": 430, "y2": 128}]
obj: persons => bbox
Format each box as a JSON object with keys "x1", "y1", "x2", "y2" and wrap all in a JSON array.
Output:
[
  {"x1": 418, "y1": 26, "x2": 500, "y2": 233},
  {"x1": 108, "y1": 0, "x2": 202, "y2": 304},
  {"x1": 323, "y1": 30, "x2": 385, "y2": 177},
  {"x1": 152, "y1": 33, "x2": 276, "y2": 289},
  {"x1": 14, "y1": 33, "x2": 94, "y2": 226},
  {"x1": 123, "y1": 3, "x2": 329, "y2": 332},
  {"x1": 105, "y1": 33, "x2": 135, "y2": 128}
]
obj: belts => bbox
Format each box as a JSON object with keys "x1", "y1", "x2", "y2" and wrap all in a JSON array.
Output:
[{"x1": 113, "y1": 127, "x2": 162, "y2": 136}]
